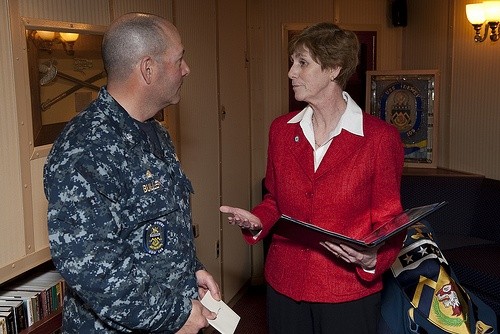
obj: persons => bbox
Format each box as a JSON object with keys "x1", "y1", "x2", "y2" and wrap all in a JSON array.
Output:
[
  {"x1": 218, "y1": 21, "x2": 409, "y2": 334},
  {"x1": 42, "y1": 11, "x2": 223, "y2": 334}
]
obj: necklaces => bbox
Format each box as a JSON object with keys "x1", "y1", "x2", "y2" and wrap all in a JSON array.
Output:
[{"x1": 315, "y1": 142, "x2": 321, "y2": 147}]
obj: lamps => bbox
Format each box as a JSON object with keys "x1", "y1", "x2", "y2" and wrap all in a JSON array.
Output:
[
  {"x1": 465, "y1": 1, "x2": 500, "y2": 43},
  {"x1": 36, "y1": 30, "x2": 80, "y2": 56}
]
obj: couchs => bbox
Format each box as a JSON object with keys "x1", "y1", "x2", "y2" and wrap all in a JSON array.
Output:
[{"x1": 401, "y1": 175, "x2": 500, "y2": 319}]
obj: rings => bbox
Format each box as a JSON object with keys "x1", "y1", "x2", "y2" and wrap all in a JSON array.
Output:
[{"x1": 336, "y1": 253, "x2": 341, "y2": 258}]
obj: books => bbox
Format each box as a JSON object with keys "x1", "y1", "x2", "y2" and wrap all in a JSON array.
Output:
[{"x1": 0, "y1": 268, "x2": 66, "y2": 334}]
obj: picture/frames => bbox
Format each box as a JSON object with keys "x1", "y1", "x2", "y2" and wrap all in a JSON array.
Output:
[
  {"x1": 366, "y1": 69, "x2": 440, "y2": 169},
  {"x1": 281, "y1": 23, "x2": 381, "y2": 115}
]
